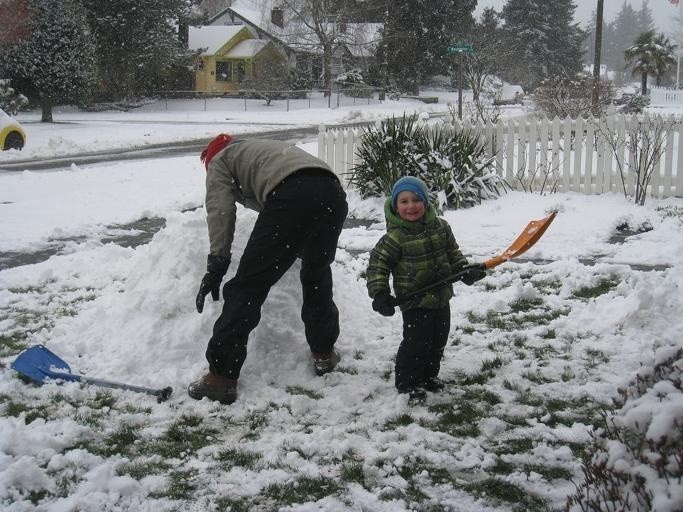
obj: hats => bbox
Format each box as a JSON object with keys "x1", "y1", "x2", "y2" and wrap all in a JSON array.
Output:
[
  {"x1": 200, "y1": 133, "x2": 232, "y2": 171},
  {"x1": 391, "y1": 176, "x2": 429, "y2": 214}
]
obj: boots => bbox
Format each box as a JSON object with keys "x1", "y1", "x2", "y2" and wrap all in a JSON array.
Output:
[
  {"x1": 313, "y1": 351, "x2": 341, "y2": 375},
  {"x1": 188, "y1": 369, "x2": 237, "y2": 405},
  {"x1": 395, "y1": 376, "x2": 445, "y2": 401}
]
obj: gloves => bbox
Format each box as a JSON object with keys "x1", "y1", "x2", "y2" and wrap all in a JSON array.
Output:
[
  {"x1": 195, "y1": 253, "x2": 231, "y2": 313},
  {"x1": 373, "y1": 292, "x2": 397, "y2": 317},
  {"x1": 457, "y1": 261, "x2": 486, "y2": 286}
]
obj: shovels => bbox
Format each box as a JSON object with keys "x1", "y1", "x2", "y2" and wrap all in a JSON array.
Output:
[
  {"x1": 371, "y1": 209, "x2": 558, "y2": 312},
  {"x1": 11, "y1": 344, "x2": 173, "y2": 404}
]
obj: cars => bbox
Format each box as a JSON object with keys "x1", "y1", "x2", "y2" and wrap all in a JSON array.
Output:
[
  {"x1": 0, "y1": 108, "x2": 27, "y2": 153},
  {"x1": 493, "y1": 86, "x2": 525, "y2": 106}
]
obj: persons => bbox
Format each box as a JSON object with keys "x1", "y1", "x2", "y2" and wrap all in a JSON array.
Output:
[
  {"x1": 186, "y1": 130, "x2": 350, "y2": 406},
  {"x1": 365, "y1": 176, "x2": 486, "y2": 406}
]
